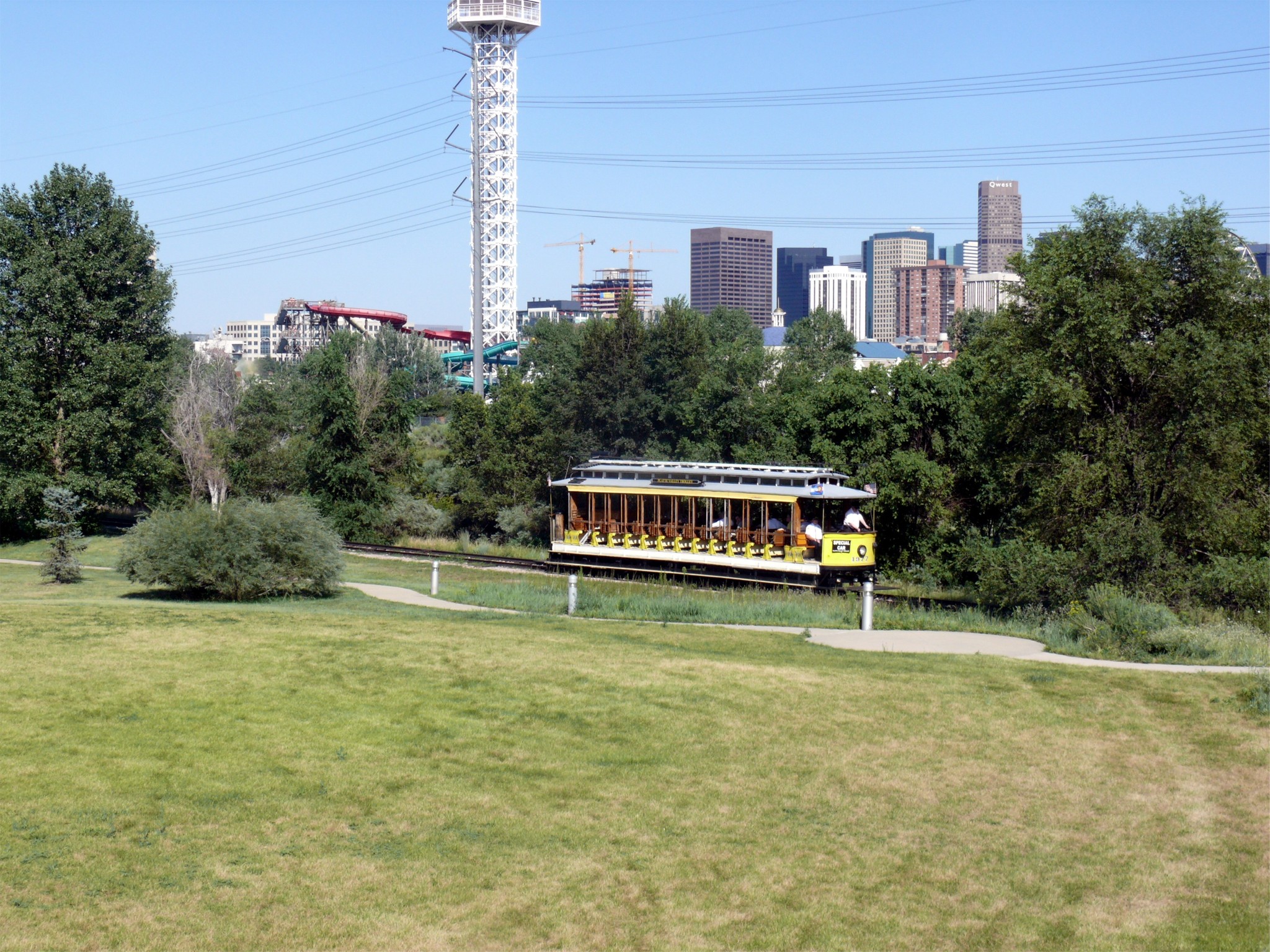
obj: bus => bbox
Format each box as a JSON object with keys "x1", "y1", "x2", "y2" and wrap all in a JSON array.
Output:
[{"x1": 547, "y1": 456, "x2": 879, "y2": 589}]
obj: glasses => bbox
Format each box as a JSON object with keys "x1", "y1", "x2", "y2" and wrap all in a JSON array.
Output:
[{"x1": 854, "y1": 507, "x2": 860, "y2": 509}]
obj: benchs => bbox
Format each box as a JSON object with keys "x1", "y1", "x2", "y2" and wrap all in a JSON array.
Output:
[
  {"x1": 792, "y1": 533, "x2": 815, "y2": 562},
  {"x1": 755, "y1": 529, "x2": 775, "y2": 560},
  {"x1": 773, "y1": 530, "x2": 793, "y2": 561},
  {"x1": 574, "y1": 517, "x2": 737, "y2": 557},
  {"x1": 737, "y1": 526, "x2": 755, "y2": 558}
]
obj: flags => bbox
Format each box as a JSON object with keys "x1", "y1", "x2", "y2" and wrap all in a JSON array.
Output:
[
  {"x1": 864, "y1": 484, "x2": 877, "y2": 494},
  {"x1": 810, "y1": 484, "x2": 823, "y2": 495}
]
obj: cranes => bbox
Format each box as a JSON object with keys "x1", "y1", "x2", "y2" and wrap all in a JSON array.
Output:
[
  {"x1": 609, "y1": 240, "x2": 678, "y2": 300},
  {"x1": 542, "y1": 233, "x2": 596, "y2": 284}
]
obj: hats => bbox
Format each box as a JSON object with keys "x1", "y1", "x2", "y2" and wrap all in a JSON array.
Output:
[{"x1": 812, "y1": 516, "x2": 820, "y2": 520}]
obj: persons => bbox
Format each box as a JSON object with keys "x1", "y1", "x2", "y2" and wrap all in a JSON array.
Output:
[
  {"x1": 805, "y1": 517, "x2": 822, "y2": 557},
  {"x1": 843, "y1": 502, "x2": 870, "y2": 532},
  {"x1": 619, "y1": 507, "x2": 844, "y2": 545}
]
